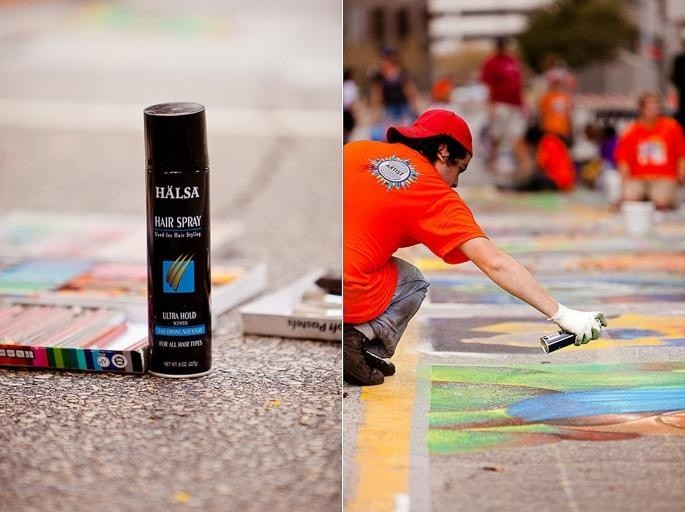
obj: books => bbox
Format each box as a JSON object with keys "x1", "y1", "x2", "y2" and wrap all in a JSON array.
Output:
[{"x1": 238, "y1": 264, "x2": 341, "y2": 341}]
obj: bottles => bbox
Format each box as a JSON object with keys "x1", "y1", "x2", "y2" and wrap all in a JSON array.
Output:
[
  {"x1": 541, "y1": 318, "x2": 604, "y2": 355},
  {"x1": 143, "y1": 98, "x2": 213, "y2": 379}
]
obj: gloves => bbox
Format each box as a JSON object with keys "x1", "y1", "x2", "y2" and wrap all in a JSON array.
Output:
[{"x1": 546, "y1": 302, "x2": 610, "y2": 348}]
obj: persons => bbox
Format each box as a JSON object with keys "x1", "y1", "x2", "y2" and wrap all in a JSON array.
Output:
[{"x1": 343, "y1": 109, "x2": 607, "y2": 385}]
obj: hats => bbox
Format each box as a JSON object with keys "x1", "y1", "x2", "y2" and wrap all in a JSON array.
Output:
[{"x1": 387, "y1": 109, "x2": 473, "y2": 157}]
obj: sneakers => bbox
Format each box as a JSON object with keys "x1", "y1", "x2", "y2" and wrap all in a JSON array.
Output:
[{"x1": 343, "y1": 326, "x2": 395, "y2": 386}]
